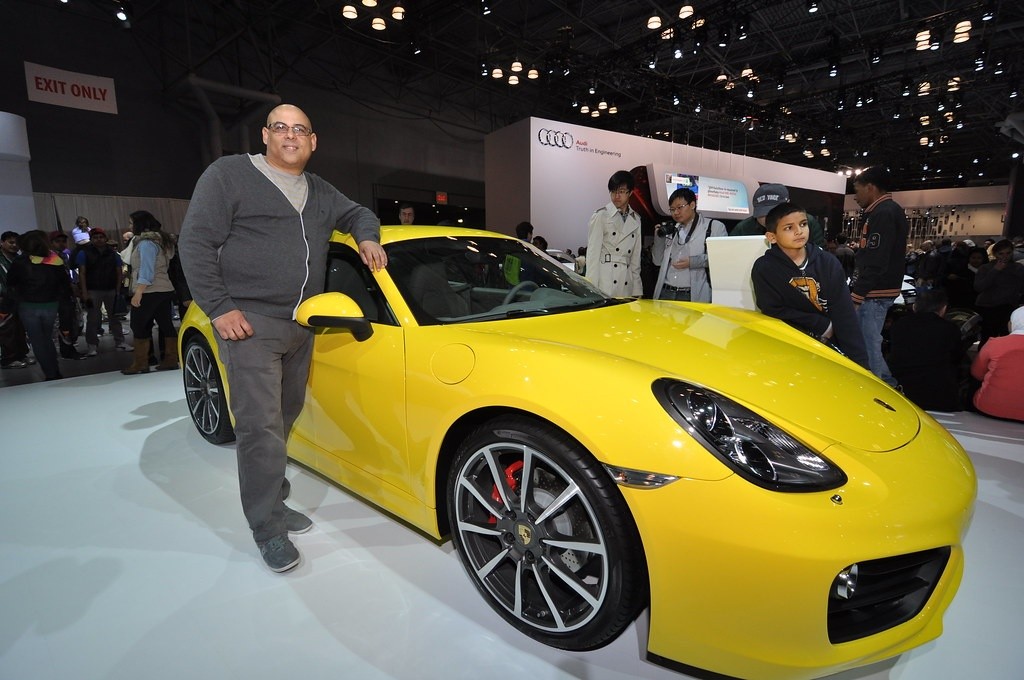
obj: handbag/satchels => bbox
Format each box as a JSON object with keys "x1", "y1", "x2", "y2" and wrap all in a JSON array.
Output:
[
  {"x1": 114, "y1": 295, "x2": 129, "y2": 315},
  {"x1": 950, "y1": 368, "x2": 981, "y2": 406}
]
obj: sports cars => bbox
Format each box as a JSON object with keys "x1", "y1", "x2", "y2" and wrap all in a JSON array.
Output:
[{"x1": 178, "y1": 222, "x2": 978, "y2": 680}]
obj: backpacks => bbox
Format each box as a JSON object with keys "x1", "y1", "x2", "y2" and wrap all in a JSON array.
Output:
[{"x1": 906, "y1": 251, "x2": 924, "y2": 278}]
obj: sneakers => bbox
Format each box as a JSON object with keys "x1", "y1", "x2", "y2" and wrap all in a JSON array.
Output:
[
  {"x1": 283, "y1": 502, "x2": 313, "y2": 533},
  {"x1": 260, "y1": 533, "x2": 301, "y2": 573},
  {"x1": 116, "y1": 343, "x2": 135, "y2": 352}
]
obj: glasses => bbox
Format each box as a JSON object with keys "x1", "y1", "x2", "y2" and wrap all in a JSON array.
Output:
[
  {"x1": 609, "y1": 190, "x2": 631, "y2": 194},
  {"x1": 267, "y1": 124, "x2": 313, "y2": 136},
  {"x1": 669, "y1": 203, "x2": 689, "y2": 212}
]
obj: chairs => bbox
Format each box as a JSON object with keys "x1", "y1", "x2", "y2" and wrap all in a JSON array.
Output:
[
  {"x1": 407, "y1": 263, "x2": 468, "y2": 321},
  {"x1": 837, "y1": 255, "x2": 855, "y2": 277},
  {"x1": 327, "y1": 259, "x2": 378, "y2": 323}
]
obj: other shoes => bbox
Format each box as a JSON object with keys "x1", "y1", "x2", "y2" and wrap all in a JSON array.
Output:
[
  {"x1": 118, "y1": 316, "x2": 126, "y2": 320},
  {"x1": 1, "y1": 360, "x2": 27, "y2": 368},
  {"x1": 61, "y1": 352, "x2": 86, "y2": 360},
  {"x1": 110, "y1": 328, "x2": 130, "y2": 335},
  {"x1": 88, "y1": 344, "x2": 98, "y2": 356},
  {"x1": 22, "y1": 356, "x2": 35, "y2": 364}
]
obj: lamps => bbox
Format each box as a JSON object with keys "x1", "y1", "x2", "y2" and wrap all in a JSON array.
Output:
[{"x1": 343, "y1": 0, "x2": 1020, "y2": 182}]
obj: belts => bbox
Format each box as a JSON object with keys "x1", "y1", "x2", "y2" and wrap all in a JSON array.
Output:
[{"x1": 663, "y1": 283, "x2": 691, "y2": 293}]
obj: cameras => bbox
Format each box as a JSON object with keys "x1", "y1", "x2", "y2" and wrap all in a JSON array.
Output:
[{"x1": 657, "y1": 222, "x2": 677, "y2": 237}]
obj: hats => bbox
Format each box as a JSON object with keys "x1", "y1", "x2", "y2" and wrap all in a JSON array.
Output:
[
  {"x1": 106, "y1": 240, "x2": 118, "y2": 244},
  {"x1": 89, "y1": 228, "x2": 109, "y2": 243},
  {"x1": 753, "y1": 184, "x2": 789, "y2": 217},
  {"x1": 963, "y1": 240, "x2": 975, "y2": 247},
  {"x1": 1011, "y1": 306, "x2": 1024, "y2": 335},
  {"x1": 48, "y1": 231, "x2": 69, "y2": 241}
]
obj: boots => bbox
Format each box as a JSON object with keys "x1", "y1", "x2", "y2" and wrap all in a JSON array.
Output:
[
  {"x1": 122, "y1": 338, "x2": 150, "y2": 375},
  {"x1": 155, "y1": 337, "x2": 178, "y2": 370}
]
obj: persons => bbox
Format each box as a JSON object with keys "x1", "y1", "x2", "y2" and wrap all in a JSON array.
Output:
[
  {"x1": 399, "y1": 202, "x2": 415, "y2": 225},
  {"x1": 0, "y1": 216, "x2": 193, "y2": 380},
  {"x1": 654, "y1": 188, "x2": 729, "y2": 302},
  {"x1": 181, "y1": 103, "x2": 388, "y2": 574},
  {"x1": 76, "y1": 226, "x2": 134, "y2": 355},
  {"x1": 517, "y1": 221, "x2": 586, "y2": 276},
  {"x1": 121, "y1": 210, "x2": 179, "y2": 375},
  {"x1": 730, "y1": 183, "x2": 824, "y2": 243},
  {"x1": 905, "y1": 235, "x2": 1024, "y2": 422},
  {"x1": 851, "y1": 166, "x2": 908, "y2": 394},
  {"x1": 826, "y1": 233, "x2": 861, "y2": 272},
  {"x1": 10, "y1": 230, "x2": 71, "y2": 379},
  {"x1": 752, "y1": 203, "x2": 875, "y2": 376},
  {"x1": 586, "y1": 171, "x2": 643, "y2": 299}
]
